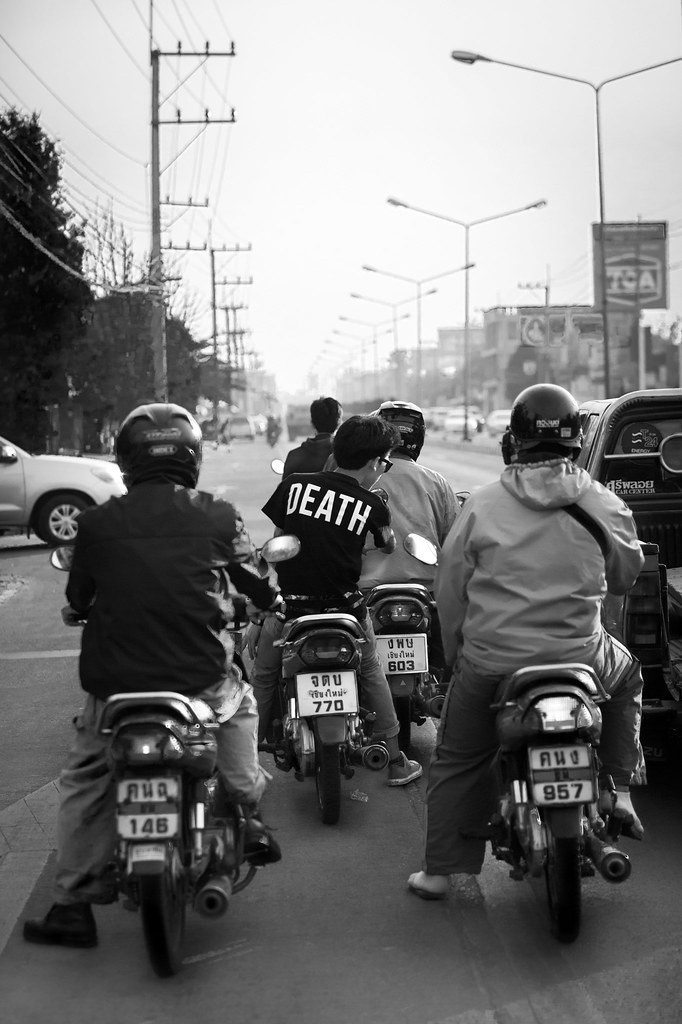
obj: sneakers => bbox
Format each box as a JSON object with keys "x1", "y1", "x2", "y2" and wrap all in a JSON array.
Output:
[{"x1": 389, "y1": 753, "x2": 423, "y2": 786}]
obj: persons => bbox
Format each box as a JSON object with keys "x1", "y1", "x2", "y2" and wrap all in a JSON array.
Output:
[
  {"x1": 408, "y1": 383, "x2": 647, "y2": 899},
  {"x1": 251, "y1": 398, "x2": 465, "y2": 786},
  {"x1": 21, "y1": 403, "x2": 280, "y2": 949},
  {"x1": 264, "y1": 414, "x2": 282, "y2": 435}
]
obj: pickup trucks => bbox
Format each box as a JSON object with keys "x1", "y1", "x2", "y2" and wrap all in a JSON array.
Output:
[{"x1": 570, "y1": 387, "x2": 682, "y2": 728}]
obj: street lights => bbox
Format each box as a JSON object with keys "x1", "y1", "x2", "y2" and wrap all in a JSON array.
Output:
[
  {"x1": 326, "y1": 314, "x2": 408, "y2": 399},
  {"x1": 452, "y1": 51, "x2": 682, "y2": 400},
  {"x1": 363, "y1": 264, "x2": 475, "y2": 405},
  {"x1": 388, "y1": 199, "x2": 547, "y2": 442},
  {"x1": 351, "y1": 291, "x2": 436, "y2": 400}
]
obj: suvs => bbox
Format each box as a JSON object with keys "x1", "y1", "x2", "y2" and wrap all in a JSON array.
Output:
[{"x1": 0, "y1": 436, "x2": 128, "y2": 545}]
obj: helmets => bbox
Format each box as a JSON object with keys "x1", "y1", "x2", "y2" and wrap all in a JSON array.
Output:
[
  {"x1": 510, "y1": 382, "x2": 582, "y2": 441},
  {"x1": 372, "y1": 401, "x2": 425, "y2": 459},
  {"x1": 115, "y1": 401, "x2": 203, "y2": 490}
]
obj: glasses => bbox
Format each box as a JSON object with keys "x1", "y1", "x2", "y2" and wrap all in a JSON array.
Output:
[{"x1": 371, "y1": 455, "x2": 392, "y2": 474}]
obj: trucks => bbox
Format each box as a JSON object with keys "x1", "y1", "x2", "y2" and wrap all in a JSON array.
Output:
[{"x1": 286, "y1": 404, "x2": 317, "y2": 441}]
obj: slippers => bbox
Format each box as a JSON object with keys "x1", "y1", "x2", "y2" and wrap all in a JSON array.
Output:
[
  {"x1": 613, "y1": 807, "x2": 644, "y2": 842},
  {"x1": 408, "y1": 870, "x2": 446, "y2": 901}
]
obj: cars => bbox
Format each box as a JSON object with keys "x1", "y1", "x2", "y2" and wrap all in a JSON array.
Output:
[
  {"x1": 225, "y1": 416, "x2": 268, "y2": 442},
  {"x1": 417, "y1": 406, "x2": 512, "y2": 440}
]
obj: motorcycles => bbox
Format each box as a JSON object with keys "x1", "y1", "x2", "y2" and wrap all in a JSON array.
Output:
[
  {"x1": 250, "y1": 487, "x2": 390, "y2": 826},
  {"x1": 400, "y1": 534, "x2": 634, "y2": 944},
  {"x1": 50, "y1": 535, "x2": 302, "y2": 979},
  {"x1": 270, "y1": 458, "x2": 471, "y2": 754},
  {"x1": 268, "y1": 424, "x2": 277, "y2": 446}
]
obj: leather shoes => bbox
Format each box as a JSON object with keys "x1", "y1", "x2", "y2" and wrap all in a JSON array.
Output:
[
  {"x1": 23, "y1": 902, "x2": 97, "y2": 950},
  {"x1": 246, "y1": 827, "x2": 281, "y2": 866}
]
